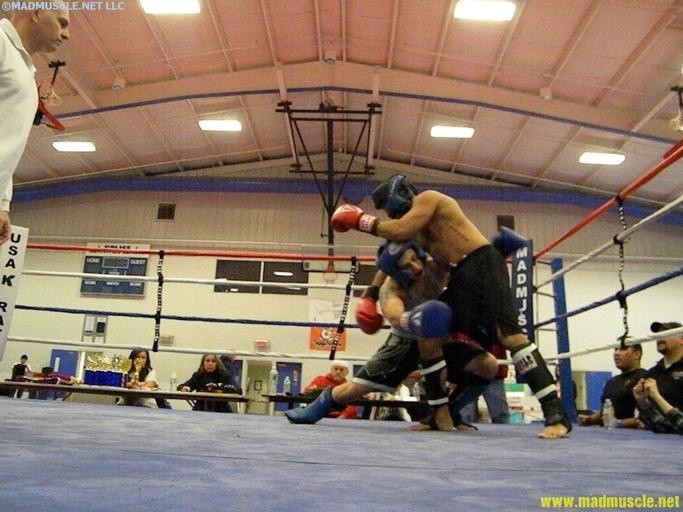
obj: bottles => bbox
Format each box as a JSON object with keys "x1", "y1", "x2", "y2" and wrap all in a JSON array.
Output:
[
  {"x1": 268, "y1": 366, "x2": 278, "y2": 395},
  {"x1": 282, "y1": 376, "x2": 290, "y2": 396},
  {"x1": 601, "y1": 398, "x2": 615, "y2": 432},
  {"x1": 168, "y1": 371, "x2": 177, "y2": 392}
]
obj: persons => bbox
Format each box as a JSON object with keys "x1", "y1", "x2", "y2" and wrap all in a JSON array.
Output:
[
  {"x1": 218, "y1": 345, "x2": 239, "y2": 413},
  {"x1": 301, "y1": 361, "x2": 360, "y2": 420},
  {"x1": 553, "y1": 364, "x2": 577, "y2": 423},
  {"x1": 329, "y1": 172, "x2": 573, "y2": 439},
  {"x1": 631, "y1": 322, "x2": 682, "y2": 429},
  {"x1": 575, "y1": 336, "x2": 648, "y2": 431},
  {"x1": 115, "y1": 348, "x2": 164, "y2": 409},
  {"x1": 459, "y1": 339, "x2": 510, "y2": 425},
  {"x1": 360, "y1": 391, "x2": 412, "y2": 423},
  {"x1": 7, "y1": 355, "x2": 32, "y2": 398},
  {"x1": 632, "y1": 377, "x2": 682, "y2": 436},
  {"x1": 284, "y1": 224, "x2": 529, "y2": 433},
  {"x1": 0, "y1": 0, "x2": 70, "y2": 247},
  {"x1": 176, "y1": 354, "x2": 242, "y2": 413}
]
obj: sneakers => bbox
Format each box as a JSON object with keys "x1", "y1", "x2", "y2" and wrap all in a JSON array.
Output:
[{"x1": 287, "y1": 406, "x2": 318, "y2": 424}]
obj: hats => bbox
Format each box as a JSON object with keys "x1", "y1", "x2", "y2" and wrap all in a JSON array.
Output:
[{"x1": 651, "y1": 322, "x2": 682, "y2": 333}]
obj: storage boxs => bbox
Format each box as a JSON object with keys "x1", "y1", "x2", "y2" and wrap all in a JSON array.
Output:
[{"x1": 84, "y1": 370, "x2": 128, "y2": 387}]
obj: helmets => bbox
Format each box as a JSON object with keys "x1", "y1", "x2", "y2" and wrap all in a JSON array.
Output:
[
  {"x1": 375, "y1": 237, "x2": 426, "y2": 282},
  {"x1": 372, "y1": 176, "x2": 416, "y2": 217}
]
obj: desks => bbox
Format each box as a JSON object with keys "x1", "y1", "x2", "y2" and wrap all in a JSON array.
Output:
[
  {"x1": 261, "y1": 394, "x2": 426, "y2": 420},
  {"x1": 2, "y1": 381, "x2": 247, "y2": 413}
]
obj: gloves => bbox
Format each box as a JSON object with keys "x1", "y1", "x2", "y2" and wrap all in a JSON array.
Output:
[
  {"x1": 399, "y1": 299, "x2": 451, "y2": 339},
  {"x1": 330, "y1": 203, "x2": 377, "y2": 235},
  {"x1": 355, "y1": 286, "x2": 383, "y2": 335},
  {"x1": 492, "y1": 225, "x2": 528, "y2": 259}
]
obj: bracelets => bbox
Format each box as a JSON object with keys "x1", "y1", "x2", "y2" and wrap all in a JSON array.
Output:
[{"x1": 588, "y1": 414, "x2": 593, "y2": 424}]
